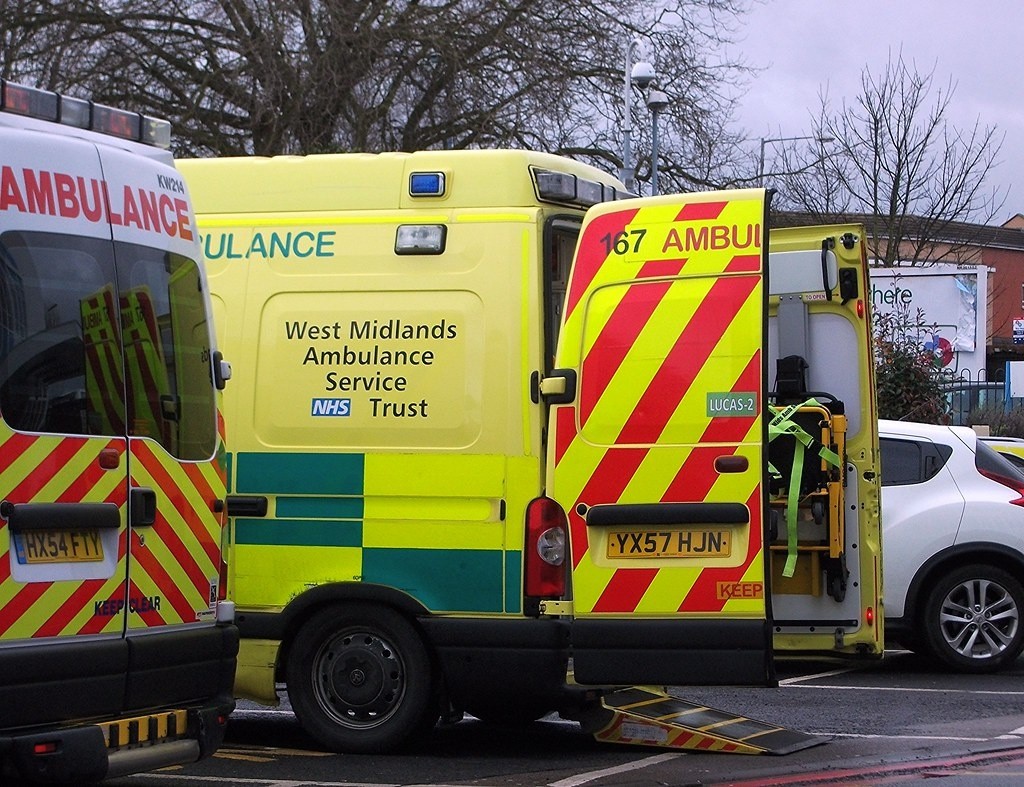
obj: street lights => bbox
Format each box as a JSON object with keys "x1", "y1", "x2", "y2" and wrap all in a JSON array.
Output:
[
  {"x1": 619, "y1": 34, "x2": 657, "y2": 184},
  {"x1": 643, "y1": 89, "x2": 670, "y2": 197},
  {"x1": 760, "y1": 133, "x2": 837, "y2": 186}
]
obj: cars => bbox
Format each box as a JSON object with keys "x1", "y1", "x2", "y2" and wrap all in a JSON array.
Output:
[
  {"x1": 874, "y1": 416, "x2": 1024, "y2": 675},
  {"x1": 975, "y1": 436, "x2": 1024, "y2": 474}
]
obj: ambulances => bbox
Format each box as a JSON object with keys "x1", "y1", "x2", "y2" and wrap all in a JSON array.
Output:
[
  {"x1": 0, "y1": 77, "x2": 239, "y2": 787},
  {"x1": 159, "y1": 145, "x2": 886, "y2": 755}
]
